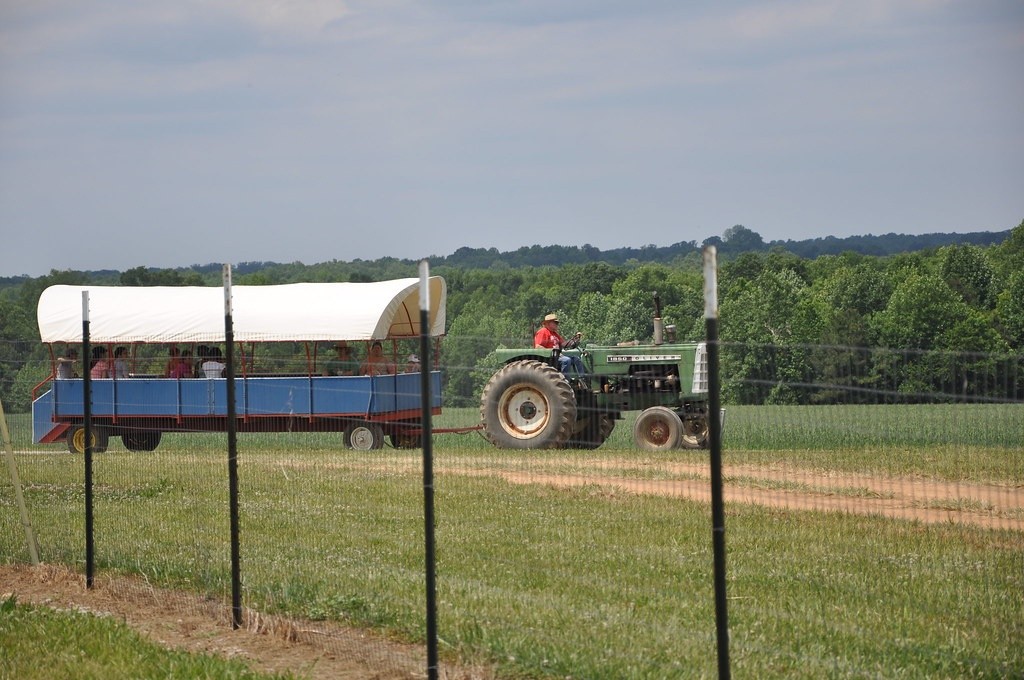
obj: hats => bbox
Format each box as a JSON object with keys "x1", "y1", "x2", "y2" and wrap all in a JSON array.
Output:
[
  {"x1": 407, "y1": 354, "x2": 420, "y2": 363},
  {"x1": 92, "y1": 346, "x2": 109, "y2": 353},
  {"x1": 64, "y1": 348, "x2": 79, "y2": 357},
  {"x1": 541, "y1": 314, "x2": 560, "y2": 325},
  {"x1": 332, "y1": 341, "x2": 355, "y2": 353}
]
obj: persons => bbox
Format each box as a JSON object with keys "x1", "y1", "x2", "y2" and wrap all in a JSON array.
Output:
[
  {"x1": 321, "y1": 341, "x2": 358, "y2": 375},
  {"x1": 110, "y1": 346, "x2": 129, "y2": 378},
  {"x1": 404, "y1": 354, "x2": 421, "y2": 372},
  {"x1": 166, "y1": 345, "x2": 227, "y2": 378},
  {"x1": 359, "y1": 342, "x2": 396, "y2": 376},
  {"x1": 534, "y1": 313, "x2": 592, "y2": 389},
  {"x1": 91, "y1": 346, "x2": 108, "y2": 378},
  {"x1": 56, "y1": 348, "x2": 79, "y2": 379}
]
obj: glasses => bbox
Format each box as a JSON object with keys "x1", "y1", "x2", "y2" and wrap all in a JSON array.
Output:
[{"x1": 553, "y1": 321, "x2": 559, "y2": 324}]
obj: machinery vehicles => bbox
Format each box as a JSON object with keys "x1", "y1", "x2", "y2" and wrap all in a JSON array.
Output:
[{"x1": 478, "y1": 293, "x2": 709, "y2": 454}]
